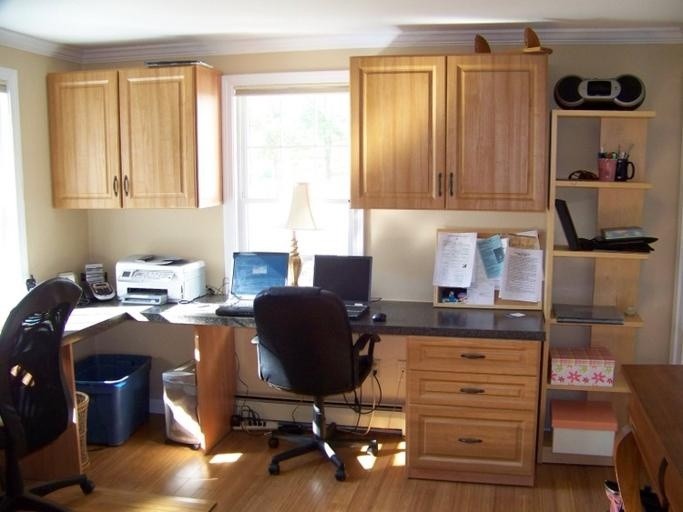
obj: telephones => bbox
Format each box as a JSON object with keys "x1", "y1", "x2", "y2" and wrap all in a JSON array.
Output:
[{"x1": 77, "y1": 263, "x2": 115, "y2": 306}]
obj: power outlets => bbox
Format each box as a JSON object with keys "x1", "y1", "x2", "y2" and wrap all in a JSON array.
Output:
[
  {"x1": 396, "y1": 360, "x2": 406, "y2": 382},
  {"x1": 368, "y1": 359, "x2": 381, "y2": 377}
]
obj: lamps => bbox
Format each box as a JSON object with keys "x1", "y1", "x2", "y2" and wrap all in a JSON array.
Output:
[{"x1": 288, "y1": 182, "x2": 317, "y2": 290}]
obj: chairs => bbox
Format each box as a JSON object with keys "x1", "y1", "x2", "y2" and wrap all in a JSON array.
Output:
[
  {"x1": 250, "y1": 288, "x2": 381, "y2": 481},
  {"x1": 0, "y1": 278, "x2": 95, "y2": 512}
]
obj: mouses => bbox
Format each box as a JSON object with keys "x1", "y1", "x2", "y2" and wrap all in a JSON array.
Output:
[{"x1": 372, "y1": 312, "x2": 386, "y2": 322}]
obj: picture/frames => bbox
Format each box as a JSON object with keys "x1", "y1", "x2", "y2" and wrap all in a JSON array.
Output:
[{"x1": 433, "y1": 225, "x2": 543, "y2": 310}]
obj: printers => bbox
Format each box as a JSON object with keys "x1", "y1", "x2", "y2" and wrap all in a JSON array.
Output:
[{"x1": 115, "y1": 254, "x2": 208, "y2": 306}]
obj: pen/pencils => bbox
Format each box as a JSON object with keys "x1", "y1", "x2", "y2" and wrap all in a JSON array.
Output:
[{"x1": 617, "y1": 143, "x2": 635, "y2": 158}]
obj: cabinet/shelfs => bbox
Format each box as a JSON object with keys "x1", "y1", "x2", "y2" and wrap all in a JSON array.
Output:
[
  {"x1": 46, "y1": 62, "x2": 226, "y2": 210},
  {"x1": 350, "y1": 52, "x2": 549, "y2": 214},
  {"x1": 537, "y1": 109, "x2": 655, "y2": 474}
]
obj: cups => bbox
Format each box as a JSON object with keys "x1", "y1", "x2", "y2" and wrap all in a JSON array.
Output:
[
  {"x1": 598, "y1": 158, "x2": 617, "y2": 181},
  {"x1": 615, "y1": 159, "x2": 634, "y2": 181}
]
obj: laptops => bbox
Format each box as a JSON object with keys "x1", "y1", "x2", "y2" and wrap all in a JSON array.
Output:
[
  {"x1": 313, "y1": 255, "x2": 373, "y2": 320},
  {"x1": 216, "y1": 252, "x2": 289, "y2": 317}
]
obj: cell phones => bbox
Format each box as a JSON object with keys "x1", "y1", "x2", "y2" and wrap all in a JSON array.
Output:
[{"x1": 90, "y1": 281, "x2": 115, "y2": 301}]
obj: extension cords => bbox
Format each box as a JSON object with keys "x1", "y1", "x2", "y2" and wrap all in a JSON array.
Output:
[{"x1": 233, "y1": 420, "x2": 279, "y2": 430}]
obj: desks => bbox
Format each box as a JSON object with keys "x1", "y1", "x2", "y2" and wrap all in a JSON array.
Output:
[
  {"x1": 613, "y1": 365, "x2": 683, "y2": 512},
  {"x1": 0, "y1": 291, "x2": 211, "y2": 479},
  {"x1": 128, "y1": 290, "x2": 547, "y2": 487}
]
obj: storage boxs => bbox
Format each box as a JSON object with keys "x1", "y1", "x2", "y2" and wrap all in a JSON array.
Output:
[
  {"x1": 74, "y1": 354, "x2": 151, "y2": 447},
  {"x1": 550, "y1": 400, "x2": 618, "y2": 457},
  {"x1": 549, "y1": 345, "x2": 615, "y2": 387}
]
